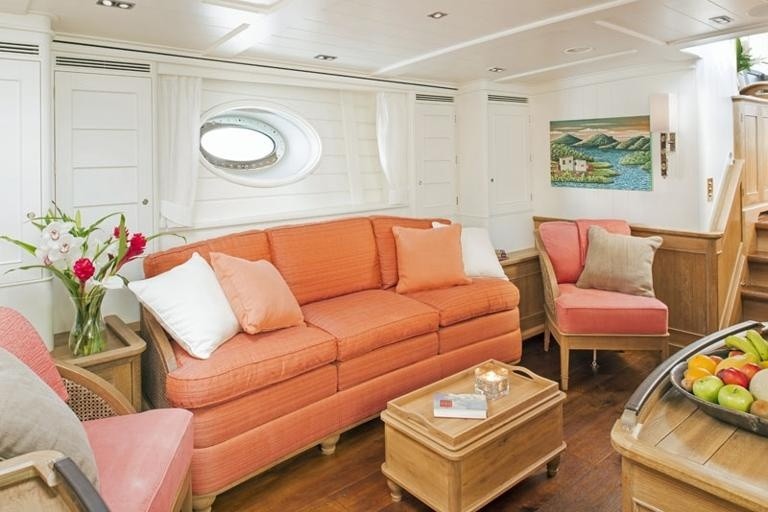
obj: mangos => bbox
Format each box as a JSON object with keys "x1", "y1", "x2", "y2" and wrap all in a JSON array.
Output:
[{"x1": 683, "y1": 352, "x2": 756, "y2": 377}]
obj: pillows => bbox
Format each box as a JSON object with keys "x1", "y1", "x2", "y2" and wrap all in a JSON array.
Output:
[
  {"x1": 576, "y1": 225, "x2": 664, "y2": 298},
  {"x1": 0, "y1": 346, "x2": 100, "y2": 496},
  {"x1": 538, "y1": 220, "x2": 632, "y2": 284},
  {"x1": 1, "y1": 307, "x2": 70, "y2": 402}
]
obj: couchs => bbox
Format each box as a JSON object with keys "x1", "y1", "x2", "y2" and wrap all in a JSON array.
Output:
[{"x1": 140, "y1": 278, "x2": 523, "y2": 512}]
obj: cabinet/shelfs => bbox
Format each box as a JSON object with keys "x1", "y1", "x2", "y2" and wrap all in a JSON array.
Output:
[
  {"x1": 734, "y1": 95, "x2": 768, "y2": 214},
  {"x1": 610, "y1": 319, "x2": 768, "y2": 512}
]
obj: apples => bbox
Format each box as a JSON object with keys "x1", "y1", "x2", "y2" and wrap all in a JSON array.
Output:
[{"x1": 693, "y1": 376, "x2": 725, "y2": 403}]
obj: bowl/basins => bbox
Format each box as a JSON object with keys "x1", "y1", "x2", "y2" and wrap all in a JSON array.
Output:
[{"x1": 670, "y1": 361, "x2": 768, "y2": 437}]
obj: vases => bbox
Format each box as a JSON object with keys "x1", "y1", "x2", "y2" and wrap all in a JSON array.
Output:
[{"x1": 68, "y1": 293, "x2": 108, "y2": 358}]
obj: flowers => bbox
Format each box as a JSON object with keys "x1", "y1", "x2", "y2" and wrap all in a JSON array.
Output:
[{"x1": 0, "y1": 201, "x2": 188, "y2": 358}]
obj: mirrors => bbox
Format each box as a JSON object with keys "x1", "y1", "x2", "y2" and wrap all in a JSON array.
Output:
[{"x1": 198, "y1": 99, "x2": 322, "y2": 189}]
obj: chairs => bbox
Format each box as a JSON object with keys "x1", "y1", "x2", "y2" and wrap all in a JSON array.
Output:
[
  {"x1": 532, "y1": 215, "x2": 671, "y2": 391},
  {"x1": 0, "y1": 359, "x2": 192, "y2": 512}
]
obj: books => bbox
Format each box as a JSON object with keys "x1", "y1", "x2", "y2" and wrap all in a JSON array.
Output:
[{"x1": 432, "y1": 392, "x2": 487, "y2": 418}]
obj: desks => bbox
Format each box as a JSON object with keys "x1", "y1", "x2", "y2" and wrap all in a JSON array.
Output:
[{"x1": 50, "y1": 316, "x2": 148, "y2": 411}]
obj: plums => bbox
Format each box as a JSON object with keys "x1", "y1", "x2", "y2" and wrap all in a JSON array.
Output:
[{"x1": 715, "y1": 363, "x2": 762, "y2": 389}]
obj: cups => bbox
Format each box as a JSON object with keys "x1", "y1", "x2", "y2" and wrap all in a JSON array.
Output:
[{"x1": 472, "y1": 362, "x2": 511, "y2": 399}]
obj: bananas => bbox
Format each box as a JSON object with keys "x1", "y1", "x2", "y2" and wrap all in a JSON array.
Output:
[{"x1": 725, "y1": 328, "x2": 768, "y2": 363}]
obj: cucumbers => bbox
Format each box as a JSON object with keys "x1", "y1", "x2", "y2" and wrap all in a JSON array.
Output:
[{"x1": 718, "y1": 383, "x2": 754, "y2": 413}]
